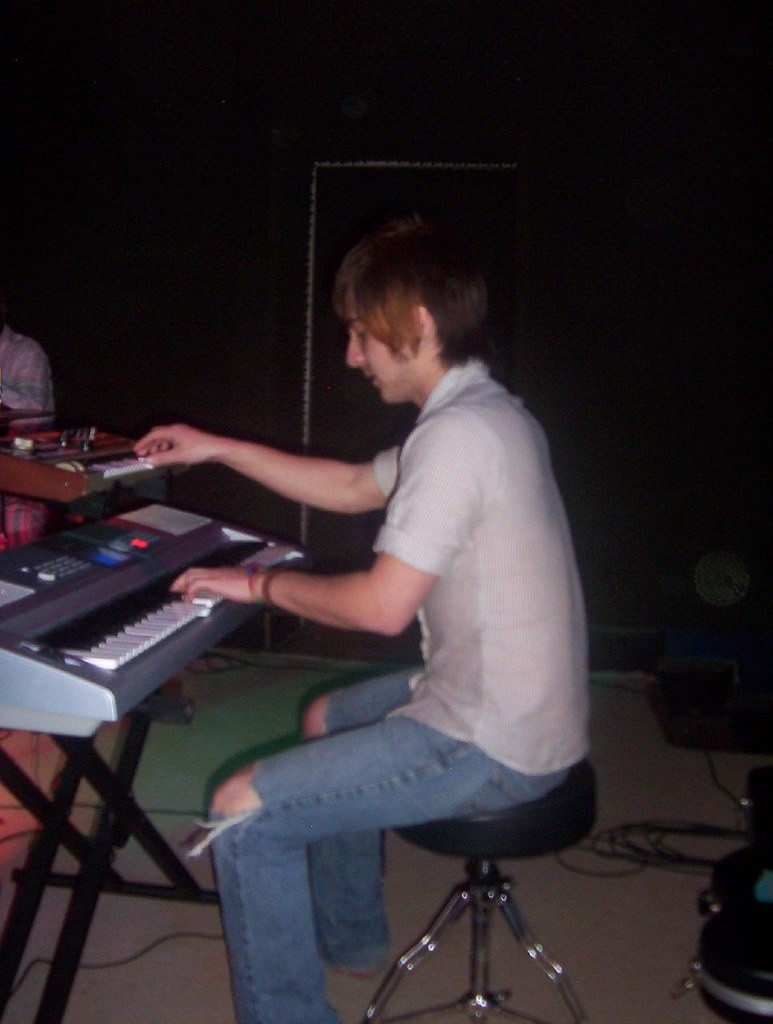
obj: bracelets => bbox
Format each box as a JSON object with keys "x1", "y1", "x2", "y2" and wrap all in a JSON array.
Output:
[
  {"x1": 241, "y1": 563, "x2": 262, "y2": 605},
  {"x1": 262, "y1": 567, "x2": 287, "y2": 608}
]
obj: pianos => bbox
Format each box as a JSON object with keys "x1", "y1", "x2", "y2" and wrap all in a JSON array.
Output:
[{"x1": 1, "y1": 502, "x2": 313, "y2": 739}]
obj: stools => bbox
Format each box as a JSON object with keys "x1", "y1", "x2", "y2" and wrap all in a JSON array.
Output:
[{"x1": 363, "y1": 757, "x2": 594, "y2": 1024}]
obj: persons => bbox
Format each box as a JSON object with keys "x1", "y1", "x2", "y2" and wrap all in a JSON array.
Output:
[
  {"x1": 0, "y1": 291, "x2": 54, "y2": 551},
  {"x1": 131, "y1": 210, "x2": 588, "y2": 1023}
]
